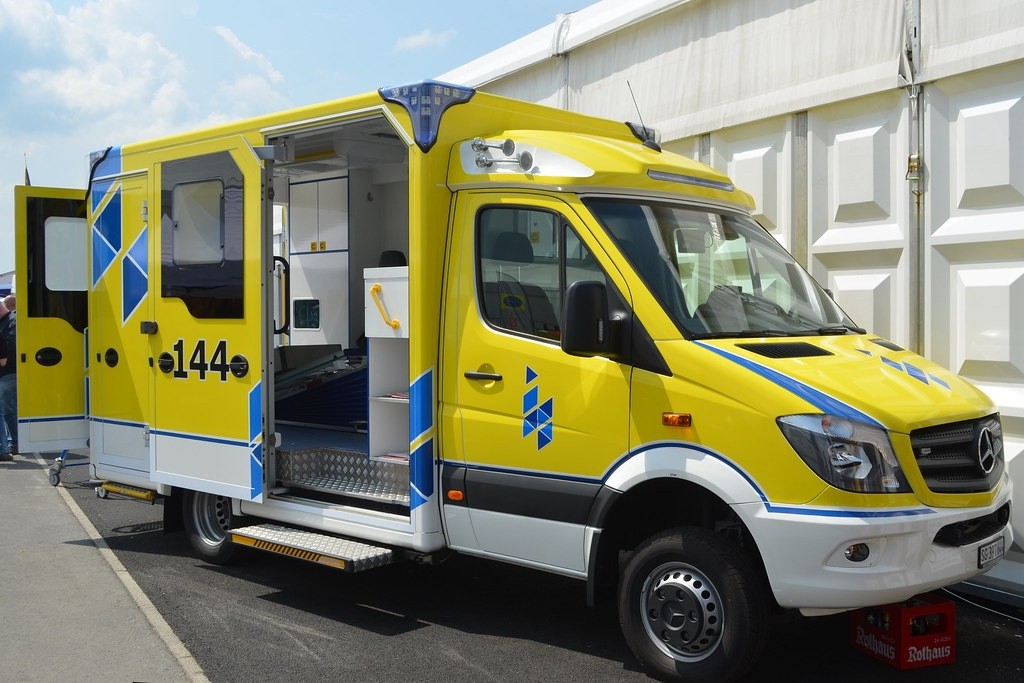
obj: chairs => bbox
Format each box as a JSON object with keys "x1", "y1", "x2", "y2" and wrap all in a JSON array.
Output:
[{"x1": 485, "y1": 231, "x2": 560, "y2": 340}]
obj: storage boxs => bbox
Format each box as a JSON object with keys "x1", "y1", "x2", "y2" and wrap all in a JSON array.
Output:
[
  {"x1": 362, "y1": 267, "x2": 410, "y2": 339},
  {"x1": 850, "y1": 595, "x2": 956, "y2": 671}
]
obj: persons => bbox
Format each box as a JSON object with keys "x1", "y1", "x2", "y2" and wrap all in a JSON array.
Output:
[{"x1": 0, "y1": 294, "x2": 29, "y2": 461}]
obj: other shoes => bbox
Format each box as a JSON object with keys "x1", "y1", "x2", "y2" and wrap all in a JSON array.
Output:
[
  {"x1": 0, "y1": 454, "x2": 13, "y2": 461},
  {"x1": 10, "y1": 447, "x2": 18, "y2": 454}
]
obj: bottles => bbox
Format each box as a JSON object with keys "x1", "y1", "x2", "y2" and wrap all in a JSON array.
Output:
[{"x1": 867, "y1": 600, "x2": 931, "y2": 635}]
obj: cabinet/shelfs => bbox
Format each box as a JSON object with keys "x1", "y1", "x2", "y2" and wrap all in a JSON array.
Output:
[{"x1": 368, "y1": 337, "x2": 409, "y2": 465}]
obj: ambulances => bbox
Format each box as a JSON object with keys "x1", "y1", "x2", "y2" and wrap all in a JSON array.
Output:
[{"x1": 14, "y1": 79, "x2": 1016, "y2": 683}]
